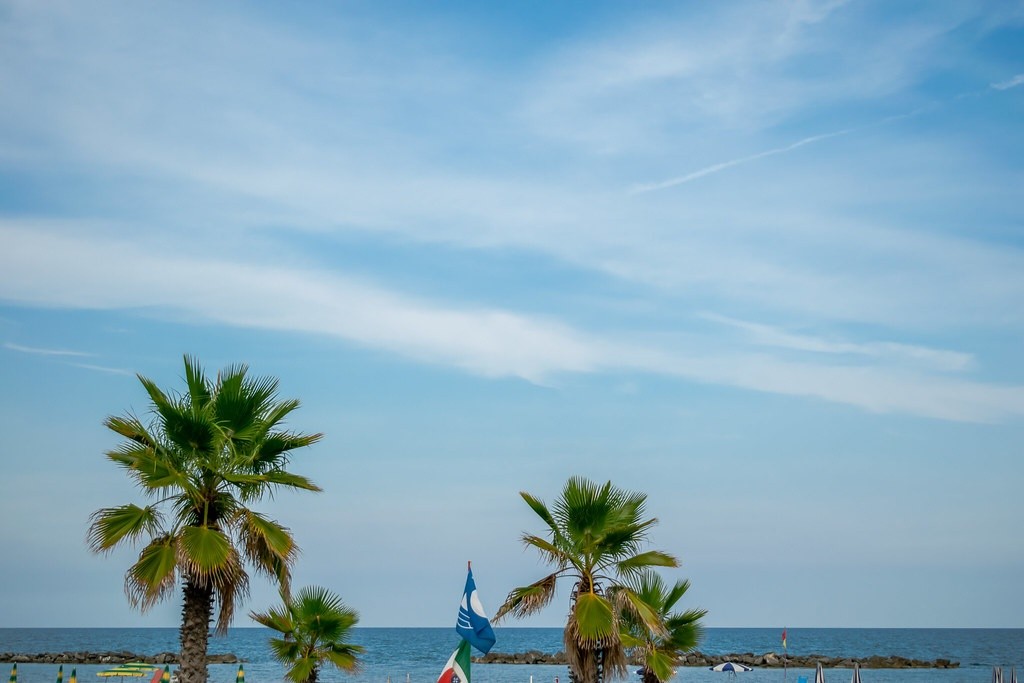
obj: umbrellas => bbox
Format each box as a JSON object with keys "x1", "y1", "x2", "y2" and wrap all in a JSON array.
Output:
[
  {"x1": 96, "y1": 660, "x2": 158, "y2": 677},
  {"x1": 709, "y1": 662, "x2": 754, "y2": 672},
  {"x1": 633, "y1": 667, "x2": 678, "y2": 675}
]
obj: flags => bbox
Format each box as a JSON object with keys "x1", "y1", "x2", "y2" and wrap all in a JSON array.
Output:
[
  {"x1": 436, "y1": 562, "x2": 496, "y2": 683},
  {"x1": 782, "y1": 627, "x2": 786, "y2": 647}
]
